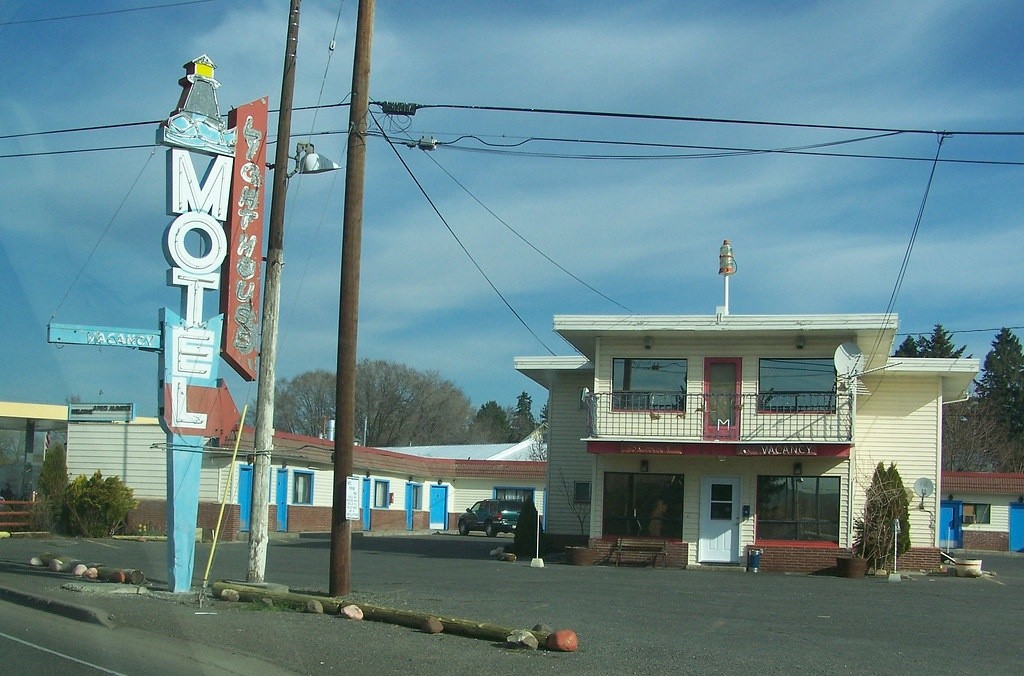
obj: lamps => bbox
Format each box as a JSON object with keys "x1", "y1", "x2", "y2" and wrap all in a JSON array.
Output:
[
  {"x1": 640, "y1": 459, "x2": 648, "y2": 472},
  {"x1": 794, "y1": 462, "x2": 802, "y2": 474}
]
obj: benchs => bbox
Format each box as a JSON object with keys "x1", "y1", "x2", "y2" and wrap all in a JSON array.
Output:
[{"x1": 614, "y1": 537, "x2": 668, "y2": 567}]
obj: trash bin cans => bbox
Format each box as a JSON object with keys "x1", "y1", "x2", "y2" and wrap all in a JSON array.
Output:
[{"x1": 747, "y1": 549, "x2": 763, "y2": 574}]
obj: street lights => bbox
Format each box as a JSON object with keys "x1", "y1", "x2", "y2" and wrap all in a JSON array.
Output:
[{"x1": 246, "y1": 143, "x2": 343, "y2": 582}]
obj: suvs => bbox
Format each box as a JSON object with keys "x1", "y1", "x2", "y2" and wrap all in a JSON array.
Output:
[{"x1": 456, "y1": 498, "x2": 523, "y2": 538}]
